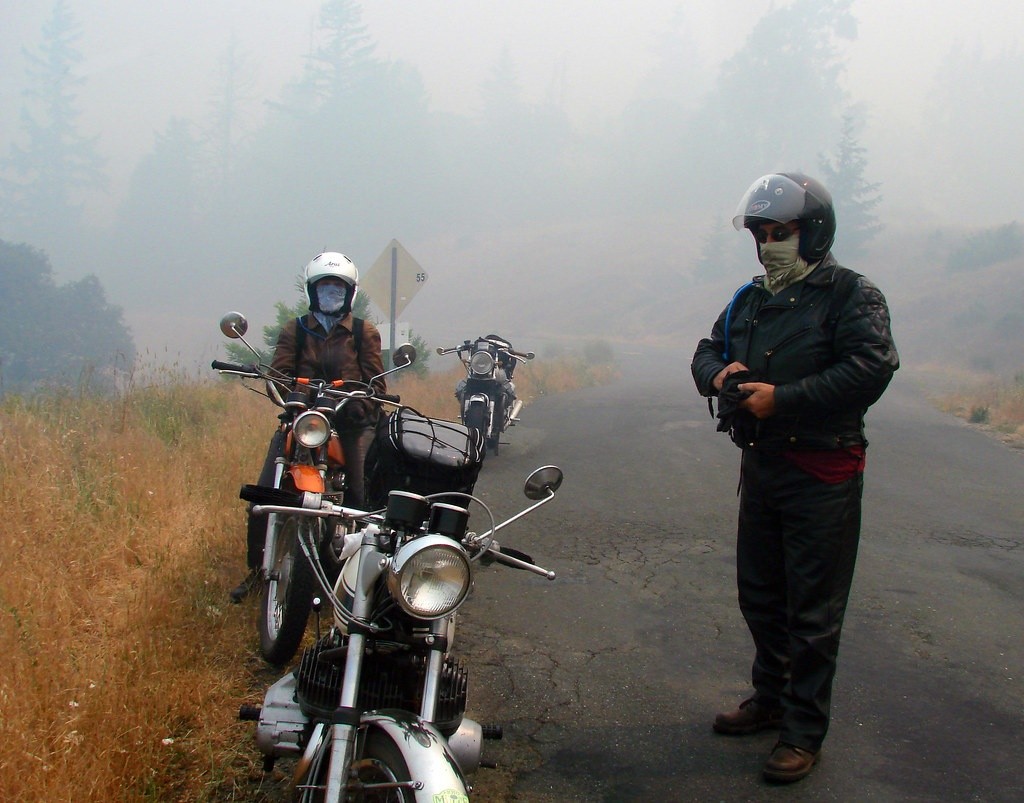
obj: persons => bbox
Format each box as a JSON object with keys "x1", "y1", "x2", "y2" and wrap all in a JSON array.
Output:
[
  {"x1": 691, "y1": 172, "x2": 900, "y2": 783},
  {"x1": 231, "y1": 252, "x2": 388, "y2": 603}
]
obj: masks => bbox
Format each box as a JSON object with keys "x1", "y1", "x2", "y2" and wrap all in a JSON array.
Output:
[{"x1": 317, "y1": 285, "x2": 346, "y2": 313}]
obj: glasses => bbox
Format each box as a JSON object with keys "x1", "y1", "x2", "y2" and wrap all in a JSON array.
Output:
[{"x1": 749, "y1": 225, "x2": 799, "y2": 243}]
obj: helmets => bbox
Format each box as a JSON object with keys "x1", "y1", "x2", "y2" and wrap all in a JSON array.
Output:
[
  {"x1": 745, "y1": 172, "x2": 837, "y2": 261},
  {"x1": 301, "y1": 250, "x2": 359, "y2": 315}
]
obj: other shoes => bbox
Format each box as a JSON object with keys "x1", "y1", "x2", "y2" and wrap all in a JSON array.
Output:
[
  {"x1": 712, "y1": 693, "x2": 784, "y2": 737},
  {"x1": 228, "y1": 570, "x2": 264, "y2": 604},
  {"x1": 760, "y1": 741, "x2": 823, "y2": 783}
]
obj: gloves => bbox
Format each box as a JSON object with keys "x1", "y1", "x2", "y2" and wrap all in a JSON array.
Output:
[{"x1": 716, "y1": 370, "x2": 767, "y2": 434}]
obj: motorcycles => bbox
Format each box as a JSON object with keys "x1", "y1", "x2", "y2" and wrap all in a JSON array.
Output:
[
  {"x1": 238, "y1": 414, "x2": 567, "y2": 803},
  {"x1": 437, "y1": 334, "x2": 535, "y2": 461},
  {"x1": 210, "y1": 310, "x2": 417, "y2": 667}
]
